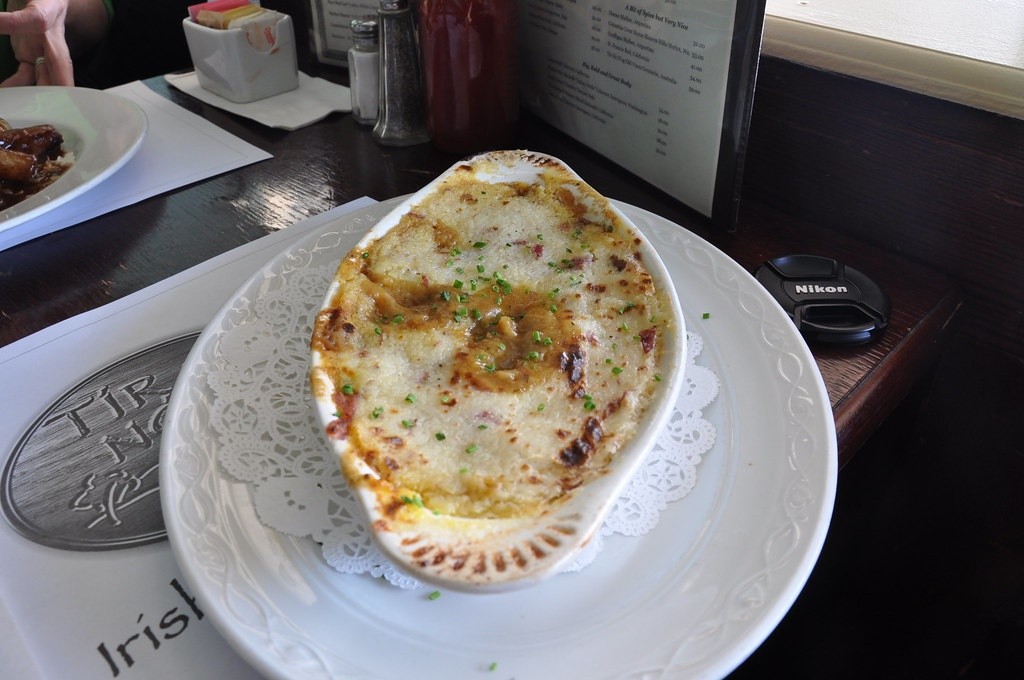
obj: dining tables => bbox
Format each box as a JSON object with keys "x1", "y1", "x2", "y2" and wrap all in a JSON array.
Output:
[{"x1": 0, "y1": 55, "x2": 962, "y2": 470}]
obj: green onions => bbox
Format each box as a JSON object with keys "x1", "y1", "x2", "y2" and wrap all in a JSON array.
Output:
[{"x1": 341, "y1": 220, "x2": 712, "y2": 510}]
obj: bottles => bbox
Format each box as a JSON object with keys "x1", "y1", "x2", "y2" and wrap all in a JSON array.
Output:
[
  {"x1": 371, "y1": 0, "x2": 430, "y2": 148},
  {"x1": 347, "y1": 14, "x2": 381, "y2": 127}
]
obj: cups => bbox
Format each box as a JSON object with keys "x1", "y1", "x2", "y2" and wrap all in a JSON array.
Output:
[{"x1": 420, "y1": 0, "x2": 521, "y2": 153}]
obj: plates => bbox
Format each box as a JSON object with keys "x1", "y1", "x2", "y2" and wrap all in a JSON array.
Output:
[
  {"x1": 0, "y1": 86, "x2": 150, "y2": 233},
  {"x1": 159, "y1": 194, "x2": 839, "y2": 680}
]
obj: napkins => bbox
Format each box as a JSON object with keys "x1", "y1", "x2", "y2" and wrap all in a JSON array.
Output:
[{"x1": 163, "y1": 69, "x2": 352, "y2": 130}]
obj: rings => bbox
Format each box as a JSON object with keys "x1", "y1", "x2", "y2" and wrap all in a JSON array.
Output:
[{"x1": 35, "y1": 57, "x2": 46, "y2": 64}]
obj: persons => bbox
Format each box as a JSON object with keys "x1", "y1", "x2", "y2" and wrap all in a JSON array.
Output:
[{"x1": 0, "y1": 0, "x2": 209, "y2": 90}]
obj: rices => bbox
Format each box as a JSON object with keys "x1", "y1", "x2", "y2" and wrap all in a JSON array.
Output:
[{"x1": 45, "y1": 150, "x2": 75, "y2": 171}]
